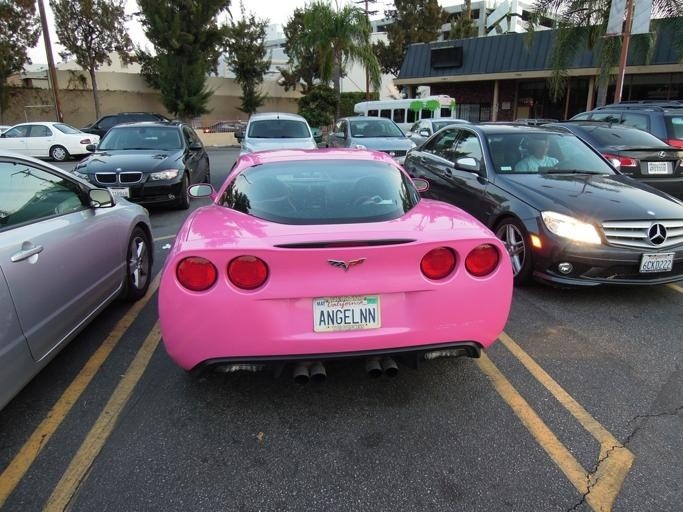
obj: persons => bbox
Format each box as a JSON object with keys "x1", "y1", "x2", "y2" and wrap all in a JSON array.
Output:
[{"x1": 513, "y1": 138, "x2": 559, "y2": 171}]
